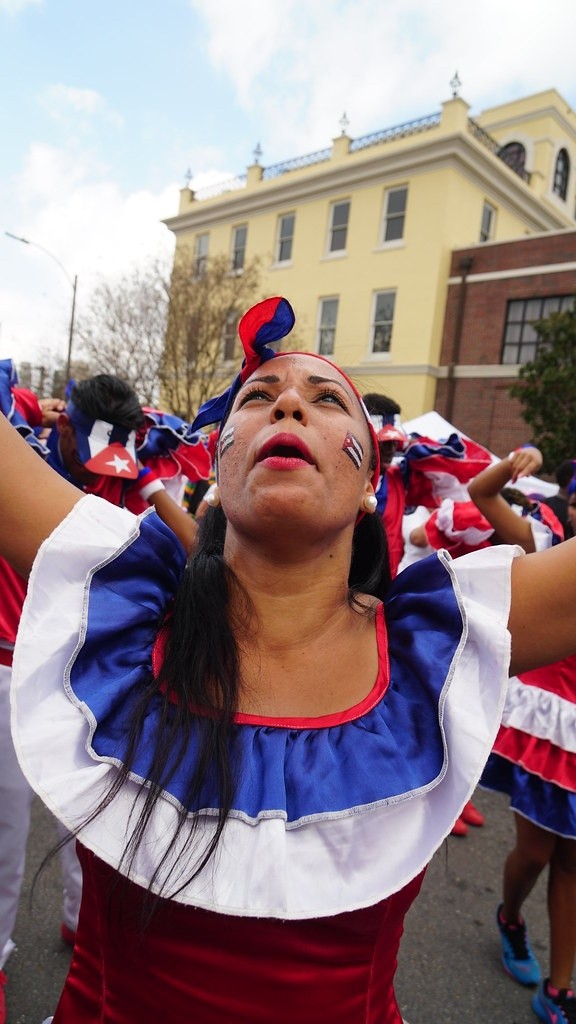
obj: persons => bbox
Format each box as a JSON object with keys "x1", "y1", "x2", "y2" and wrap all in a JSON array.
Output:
[{"x1": 0, "y1": 352, "x2": 576, "y2": 1024}]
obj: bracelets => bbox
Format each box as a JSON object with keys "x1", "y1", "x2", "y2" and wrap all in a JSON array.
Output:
[
  {"x1": 132, "y1": 467, "x2": 165, "y2": 502},
  {"x1": 509, "y1": 451, "x2": 514, "y2": 461}
]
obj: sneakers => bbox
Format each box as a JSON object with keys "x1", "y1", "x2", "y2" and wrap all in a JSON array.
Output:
[
  {"x1": 495, "y1": 903, "x2": 542, "y2": 984},
  {"x1": 533, "y1": 977, "x2": 576, "y2": 1024}
]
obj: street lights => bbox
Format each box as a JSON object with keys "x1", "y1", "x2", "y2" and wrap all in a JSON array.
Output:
[{"x1": 4, "y1": 231, "x2": 76, "y2": 402}]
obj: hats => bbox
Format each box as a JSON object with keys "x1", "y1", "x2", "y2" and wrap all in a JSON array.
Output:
[
  {"x1": 369, "y1": 414, "x2": 409, "y2": 444},
  {"x1": 67, "y1": 398, "x2": 139, "y2": 479}
]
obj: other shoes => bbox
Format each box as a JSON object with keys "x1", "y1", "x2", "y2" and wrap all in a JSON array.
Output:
[
  {"x1": 0, "y1": 971, "x2": 8, "y2": 1024},
  {"x1": 60, "y1": 920, "x2": 76, "y2": 943},
  {"x1": 451, "y1": 801, "x2": 485, "y2": 835}
]
